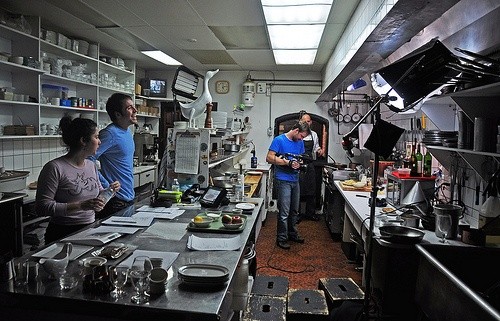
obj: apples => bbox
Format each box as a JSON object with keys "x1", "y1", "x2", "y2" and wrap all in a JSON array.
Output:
[
  {"x1": 194, "y1": 216, "x2": 203, "y2": 222},
  {"x1": 222, "y1": 215, "x2": 242, "y2": 224}
]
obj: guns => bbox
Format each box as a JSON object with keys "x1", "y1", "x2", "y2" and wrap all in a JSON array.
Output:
[
  {"x1": 341, "y1": 137, "x2": 355, "y2": 158},
  {"x1": 281, "y1": 152, "x2": 347, "y2": 175}
]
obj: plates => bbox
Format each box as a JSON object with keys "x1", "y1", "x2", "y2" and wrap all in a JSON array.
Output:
[
  {"x1": 236, "y1": 202, "x2": 255, "y2": 213},
  {"x1": 178, "y1": 263, "x2": 229, "y2": 288},
  {"x1": 422, "y1": 129, "x2": 459, "y2": 145},
  {"x1": 40, "y1": 29, "x2": 132, "y2": 70}
]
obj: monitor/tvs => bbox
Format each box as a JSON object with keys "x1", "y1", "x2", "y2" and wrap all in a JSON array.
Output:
[
  {"x1": 139, "y1": 78, "x2": 167, "y2": 97},
  {"x1": 199, "y1": 186, "x2": 227, "y2": 208}
]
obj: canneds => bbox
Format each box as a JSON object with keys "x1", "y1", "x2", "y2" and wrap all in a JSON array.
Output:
[{"x1": 70, "y1": 97, "x2": 83, "y2": 108}]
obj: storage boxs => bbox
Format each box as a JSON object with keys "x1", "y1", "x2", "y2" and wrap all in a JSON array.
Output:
[{"x1": 136, "y1": 105, "x2": 159, "y2": 116}]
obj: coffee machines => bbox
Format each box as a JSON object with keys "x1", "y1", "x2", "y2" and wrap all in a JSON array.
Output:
[{"x1": 134, "y1": 133, "x2": 160, "y2": 166}]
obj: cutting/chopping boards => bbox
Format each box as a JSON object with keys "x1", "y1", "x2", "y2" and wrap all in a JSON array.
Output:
[{"x1": 339, "y1": 180, "x2": 383, "y2": 191}]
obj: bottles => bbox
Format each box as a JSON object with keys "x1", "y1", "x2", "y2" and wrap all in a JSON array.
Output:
[
  {"x1": 171, "y1": 178, "x2": 180, "y2": 192},
  {"x1": 391, "y1": 144, "x2": 432, "y2": 177},
  {"x1": 40, "y1": 84, "x2": 96, "y2": 109},
  {"x1": 479, "y1": 190, "x2": 500, "y2": 244}
]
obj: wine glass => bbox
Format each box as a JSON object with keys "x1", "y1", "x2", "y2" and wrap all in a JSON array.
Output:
[
  {"x1": 131, "y1": 256, "x2": 153, "y2": 304},
  {"x1": 109, "y1": 265, "x2": 129, "y2": 297}
]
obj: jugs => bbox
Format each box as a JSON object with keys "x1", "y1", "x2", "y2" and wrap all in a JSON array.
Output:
[{"x1": 39, "y1": 242, "x2": 71, "y2": 274}]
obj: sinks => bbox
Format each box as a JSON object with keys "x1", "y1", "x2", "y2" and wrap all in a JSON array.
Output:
[{"x1": 0, "y1": 169, "x2": 29, "y2": 192}]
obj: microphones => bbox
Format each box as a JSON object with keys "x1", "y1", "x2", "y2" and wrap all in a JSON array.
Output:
[{"x1": 284, "y1": 152, "x2": 311, "y2": 161}]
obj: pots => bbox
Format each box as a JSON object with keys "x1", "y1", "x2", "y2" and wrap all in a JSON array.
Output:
[
  {"x1": 327, "y1": 101, "x2": 362, "y2": 170},
  {"x1": 379, "y1": 203, "x2": 486, "y2": 246}
]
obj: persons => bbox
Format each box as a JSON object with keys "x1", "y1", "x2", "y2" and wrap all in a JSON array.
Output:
[
  {"x1": 266, "y1": 121, "x2": 312, "y2": 250},
  {"x1": 296, "y1": 114, "x2": 323, "y2": 224},
  {"x1": 86, "y1": 93, "x2": 138, "y2": 220},
  {"x1": 35, "y1": 116, "x2": 121, "y2": 245}
]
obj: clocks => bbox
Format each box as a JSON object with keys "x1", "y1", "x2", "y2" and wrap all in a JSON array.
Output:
[{"x1": 215, "y1": 80, "x2": 230, "y2": 94}]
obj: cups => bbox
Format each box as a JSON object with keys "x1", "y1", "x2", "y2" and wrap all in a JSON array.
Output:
[
  {"x1": 11, "y1": 255, "x2": 168, "y2": 298},
  {"x1": 250, "y1": 156, "x2": 257, "y2": 168},
  {"x1": 0, "y1": 51, "x2": 36, "y2": 103},
  {"x1": 40, "y1": 51, "x2": 134, "y2": 92}
]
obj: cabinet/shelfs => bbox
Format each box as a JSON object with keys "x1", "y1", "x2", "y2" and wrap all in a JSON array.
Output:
[
  {"x1": 0, "y1": 15, "x2": 136, "y2": 141},
  {"x1": 137, "y1": 93, "x2": 161, "y2": 165},
  {"x1": 133, "y1": 170, "x2": 157, "y2": 203},
  {"x1": 167, "y1": 129, "x2": 250, "y2": 189},
  {"x1": 421, "y1": 82, "x2": 500, "y2": 184}
]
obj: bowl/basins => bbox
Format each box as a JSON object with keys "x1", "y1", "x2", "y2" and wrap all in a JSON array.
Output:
[
  {"x1": 40, "y1": 124, "x2": 63, "y2": 136},
  {"x1": 191, "y1": 208, "x2": 243, "y2": 230},
  {"x1": 212, "y1": 176, "x2": 236, "y2": 189},
  {"x1": 223, "y1": 143, "x2": 241, "y2": 151},
  {"x1": 174, "y1": 122, "x2": 186, "y2": 130}
]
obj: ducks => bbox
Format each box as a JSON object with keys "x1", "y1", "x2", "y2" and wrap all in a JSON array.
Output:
[{"x1": 178, "y1": 68, "x2": 221, "y2": 130}]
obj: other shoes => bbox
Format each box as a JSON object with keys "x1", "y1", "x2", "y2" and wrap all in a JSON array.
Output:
[
  {"x1": 292, "y1": 235, "x2": 304, "y2": 243},
  {"x1": 296, "y1": 216, "x2": 301, "y2": 224},
  {"x1": 307, "y1": 214, "x2": 319, "y2": 221},
  {"x1": 277, "y1": 241, "x2": 290, "y2": 249}
]
